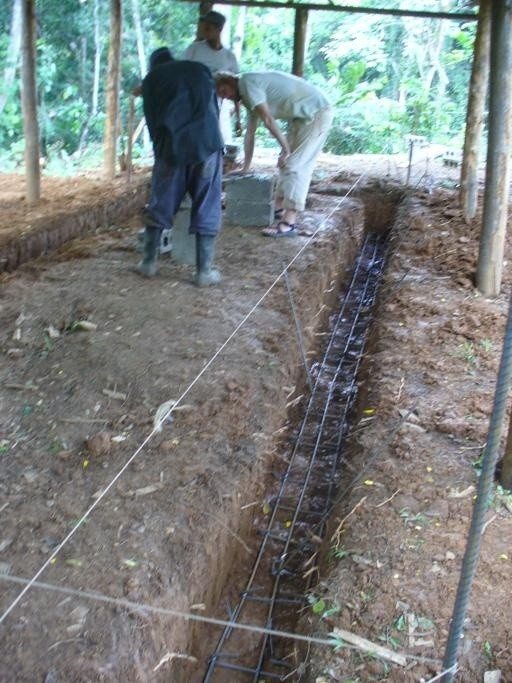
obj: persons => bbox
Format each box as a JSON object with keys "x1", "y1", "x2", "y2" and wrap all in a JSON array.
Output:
[
  {"x1": 214, "y1": 69, "x2": 333, "y2": 238},
  {"x1": 138, "y1": 47, "x2": 222, "y2": 289},
  {"x1": 132, "y1": 10, "x2": 244, "y2": 138}
]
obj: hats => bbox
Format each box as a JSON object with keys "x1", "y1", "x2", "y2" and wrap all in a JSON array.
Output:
[{"x1": 199, "y1": 10, "x2": 226, "y2": 28}]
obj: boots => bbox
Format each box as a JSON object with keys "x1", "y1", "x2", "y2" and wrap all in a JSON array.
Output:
[
  {"x1": 192, "y1": 233, "x2": 222, "y2": 288},
  {"x1": 136, "y1": 221, "x2": 164, "y2": 280}
]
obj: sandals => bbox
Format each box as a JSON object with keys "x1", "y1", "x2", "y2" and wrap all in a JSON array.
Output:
[
  {"x1": 272, "y1": 207, "x2": 285, "y2": 219},
  {"x1": 262, "y1": 220, "x2": 300, "y2": 238}
]
